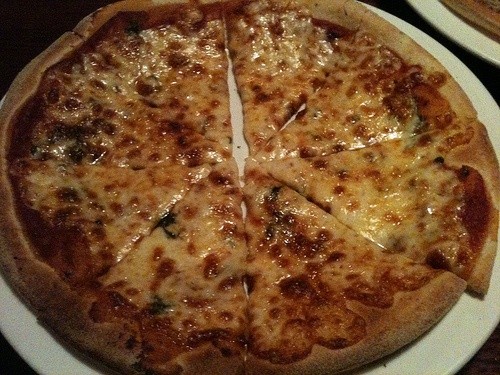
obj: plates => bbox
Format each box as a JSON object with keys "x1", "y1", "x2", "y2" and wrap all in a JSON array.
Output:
[
  {"x1": 0, "y1": 1, "x2": 498, "y2": 374},
  {"x1": 406, "y1": 0, "x2": 500, "y2": 68}
]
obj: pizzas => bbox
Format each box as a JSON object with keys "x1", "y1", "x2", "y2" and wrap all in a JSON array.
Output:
[{"x1": 0, "y1": 0, "x2": 500, "y2": 375}]
